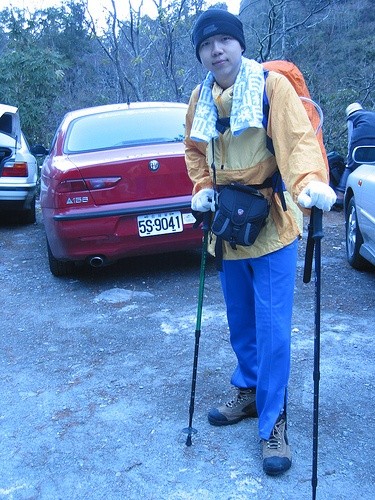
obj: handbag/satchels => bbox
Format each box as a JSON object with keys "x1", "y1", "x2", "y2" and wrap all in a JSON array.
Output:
[{"x1": 210, "y1": 181, "x2": 268, "y2": 247}]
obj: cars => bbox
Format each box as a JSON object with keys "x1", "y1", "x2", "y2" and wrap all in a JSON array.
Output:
[
  {"x1": 343, "y1": 145, "x2": 375, "y2": 267},
  {"x1": 0, "y1": 103, "x2": 39, "y2": 224},
  {"x1": 30, "y1": 95, "x2": 216, "y2": 278}
]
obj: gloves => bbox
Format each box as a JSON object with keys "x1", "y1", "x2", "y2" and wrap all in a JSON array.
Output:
[
  {"x1": 297, "y1": 181, "x2": 336, "y2": 212},
  {"x1": 191, "y1": 188, "x2": 219, "y2": 213}
]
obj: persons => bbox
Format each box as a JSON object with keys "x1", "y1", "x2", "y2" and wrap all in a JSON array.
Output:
[
  {"x1": 183, "y1": 9, "x2": 336, "y2": 475},
  {"x1": 334, "y1": 102, "x2": 375, "y2": 205}
]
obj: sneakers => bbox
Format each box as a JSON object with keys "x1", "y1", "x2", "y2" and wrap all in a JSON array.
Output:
[
  {"x1": 258, "y1": 415, "x2": 292, "y2": 476},
  {"x1": 208, "y1": 388, "x2": 258, "y2": 426}
]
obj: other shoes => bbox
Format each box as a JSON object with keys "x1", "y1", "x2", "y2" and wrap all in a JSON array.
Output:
[{"x1": 331, "y1": 190, "x2": 344, "y2": 212}]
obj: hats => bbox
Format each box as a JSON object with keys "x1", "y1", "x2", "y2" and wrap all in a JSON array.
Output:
[
  {"x1": 192, "y1": 8, "x2": 246, "y2": 64},
  {"x1": 345, "y1": 103, "x2": 362, "y2": 116}
]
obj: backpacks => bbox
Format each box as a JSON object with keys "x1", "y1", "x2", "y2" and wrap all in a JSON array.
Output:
[
  {"x1": 327, "y1": 151, "x2": 346, "y2": 186},
  {"x1": 215, "y1": 61, "x2": 329, "y2": 188}
]
